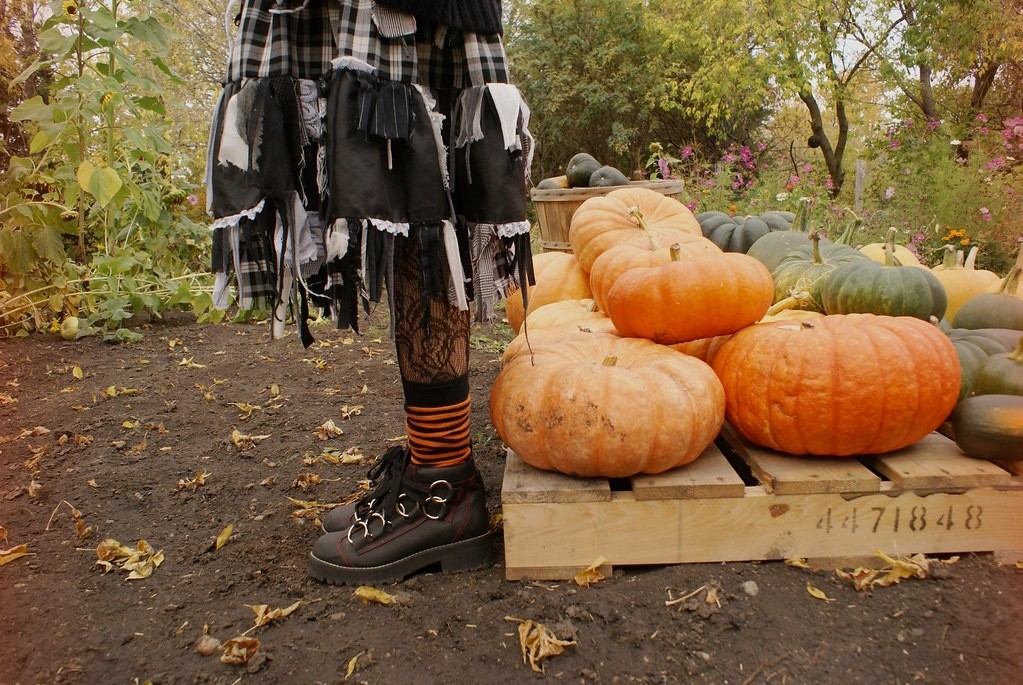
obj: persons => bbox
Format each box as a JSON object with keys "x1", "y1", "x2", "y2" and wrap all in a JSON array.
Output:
[{"x1": 203, "y1": 0, "x2": 538, "y2": 588}]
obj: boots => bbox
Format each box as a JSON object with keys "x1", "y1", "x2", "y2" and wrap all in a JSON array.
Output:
[{"x1": 307, "y1": 445, "x2": 494, "y2": 586}]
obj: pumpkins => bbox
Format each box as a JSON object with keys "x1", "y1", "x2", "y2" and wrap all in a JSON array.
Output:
[
  {"x1": 491, "y1": 153, "x2": 1023, "y2": 478},
  {"x1": 62, "y1": 317, "x2": 80, "y2": 338}
]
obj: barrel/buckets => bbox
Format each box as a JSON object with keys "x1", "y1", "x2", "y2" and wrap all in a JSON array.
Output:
[{"x1": 529, "y1": 179, "x2": 684, "y2": 254}]
